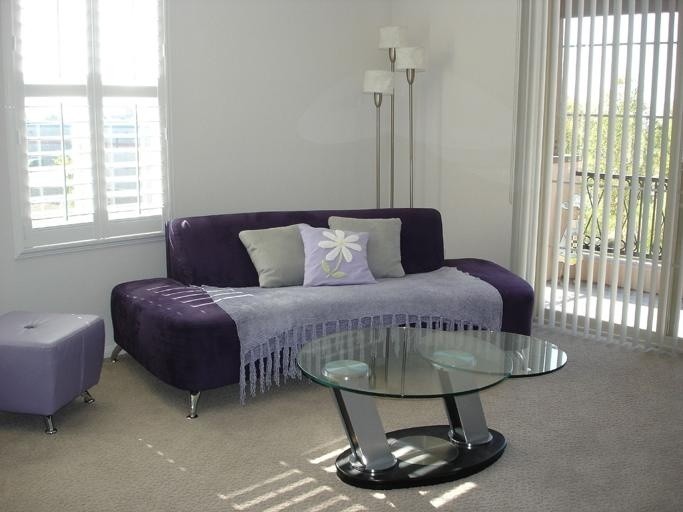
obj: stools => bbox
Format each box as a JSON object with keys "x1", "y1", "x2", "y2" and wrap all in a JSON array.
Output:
[{"x1": 0, "y1": 310, "x2": 105, "y2": 434}]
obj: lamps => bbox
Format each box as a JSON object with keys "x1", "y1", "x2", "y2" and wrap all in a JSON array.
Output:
[
  {"x1": 379, "y1": 26, "x2": 408, "y2": 208},
  {"x1": 395, "y1": 48, "x2": 426, "y2": 208},
  {"x1": 363, "y1": 70, "x2": 394, "y2": 209}
]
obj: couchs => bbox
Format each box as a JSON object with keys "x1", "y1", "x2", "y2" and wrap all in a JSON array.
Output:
[{"x1": 111, "y1": 208, "x2": 534, "y2": 419}]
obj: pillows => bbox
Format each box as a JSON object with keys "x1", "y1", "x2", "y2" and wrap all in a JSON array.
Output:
[
  {"x1": 298, "y1": 223, "x2": 376, "y2": 287},
  {"x1": 328, "y1": 216, "x2": 405, "y2": 278},
  {"x1": 239, "y1": 223, "x2": 304, "y2": 288}
]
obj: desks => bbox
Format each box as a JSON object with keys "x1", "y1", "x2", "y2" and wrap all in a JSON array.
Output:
[{"x1": 297, "y1": 327, "x2": 568, "y2": 490}]
